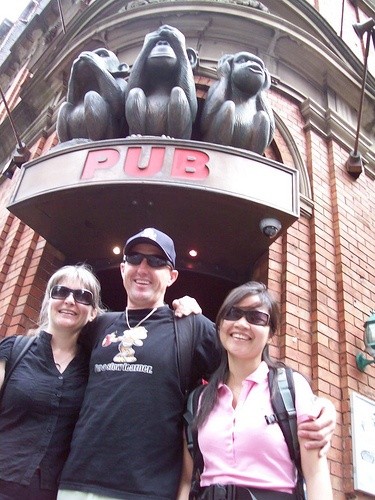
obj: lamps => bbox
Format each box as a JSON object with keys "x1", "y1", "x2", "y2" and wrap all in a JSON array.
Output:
[
  {"x1": 347, "y1": 17, "x2": 375, "y2": 179},
  {"x1": 356, "y1": 314, "x2": 375, "y2": 372}
]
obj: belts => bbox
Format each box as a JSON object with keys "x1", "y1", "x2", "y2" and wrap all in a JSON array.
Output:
[{"x1": 198, "y1": 485, "x2": 296, "y2": 500}]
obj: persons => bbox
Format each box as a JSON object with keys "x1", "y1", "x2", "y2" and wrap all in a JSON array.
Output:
[
  {"x1": 0, "y1": 265, "x2": 202, "y2": 500},
  {"x1": 177, "y1": 282, "x2": 334, "y2": 500},
  {"x1": 57, "y1": 228, "x2": 336, "y2": 500}
]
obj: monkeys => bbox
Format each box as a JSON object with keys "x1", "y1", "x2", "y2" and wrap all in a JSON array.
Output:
[
  {"x1": 56, "y1": 48, "x2": 130, "y2": 141},
  {"x1": 197, "y1": 51, "x2": 275, "y2": 158},
  {"x1": 125, "y1": 24, "x2": 200, "y2": 140}
]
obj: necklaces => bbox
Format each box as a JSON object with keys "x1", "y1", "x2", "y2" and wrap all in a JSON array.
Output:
[{"x1": 125, "y1": 307, "x2": 157, "y2": 329}]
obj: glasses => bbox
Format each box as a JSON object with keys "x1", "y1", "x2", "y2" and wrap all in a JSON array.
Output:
[
  {"x1": 124, "y1": 252, "x2": 173, "y2": 270},
  {"x1": 224, "y1": 308, "x2": 271, "y2": 326},
  {"x1": 48, "y1": 285, "x2": 95, "y2": 307}
]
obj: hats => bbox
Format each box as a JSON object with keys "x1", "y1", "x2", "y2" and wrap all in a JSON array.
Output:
[{"x1": 123, "y1": 228, "x2": 176, "y2": 269}]
